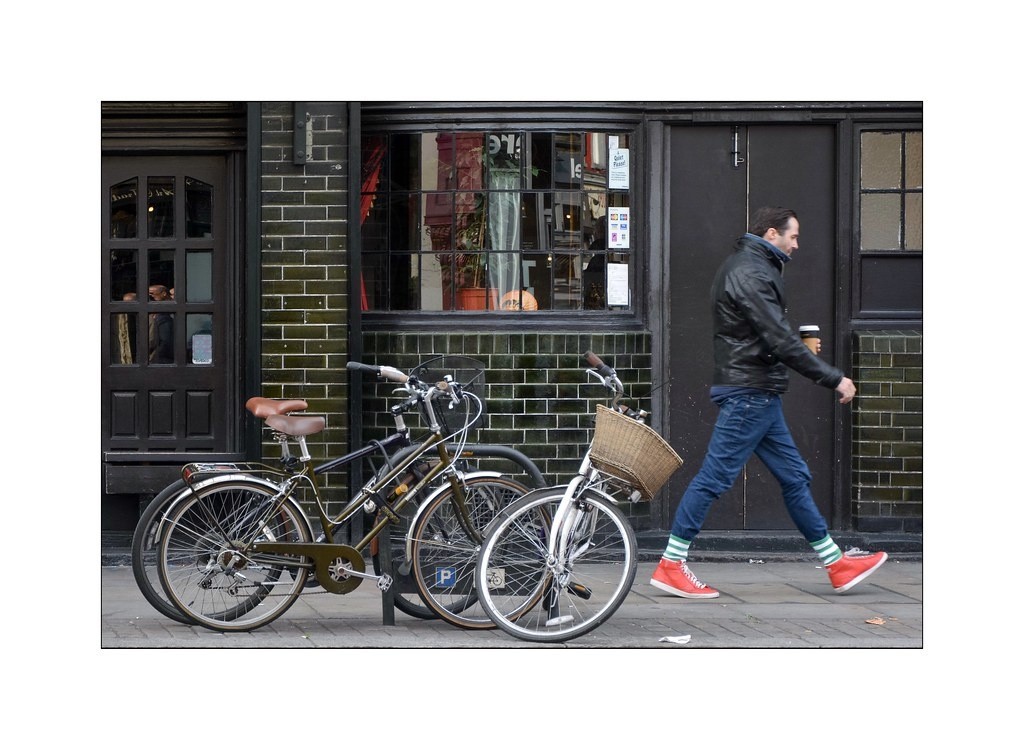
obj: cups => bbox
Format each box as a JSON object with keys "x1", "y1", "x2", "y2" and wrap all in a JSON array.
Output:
[{"x1": 798, "y1": 325, "x2": 822, "y2": 354}]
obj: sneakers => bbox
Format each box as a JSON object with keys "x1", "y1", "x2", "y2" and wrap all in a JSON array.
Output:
[
  {"x1": 825, "y1": 551, "x2": 889, "y2": 594},
  {"x1": 650, "y1": 556, "x2": 719, "y2": 599}
]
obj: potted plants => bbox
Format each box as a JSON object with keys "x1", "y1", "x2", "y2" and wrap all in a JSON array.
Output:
[{"x1": 424, "y1": 191, "x2": 516, "y2": 311}]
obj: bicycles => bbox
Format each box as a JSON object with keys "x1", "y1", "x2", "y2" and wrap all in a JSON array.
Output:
[
  {"x1": 475, "y1": 350, "x2": 684, "y2": 640},
  {"x1": 130, "y1": 362, "x2": 553, "y2": 631}
]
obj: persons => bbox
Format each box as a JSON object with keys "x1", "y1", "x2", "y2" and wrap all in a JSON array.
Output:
[
  {"x1": 584, "y1": 216, "x2": 606, "y2": 251},
  {"x1": 113, "y1": 285, "x2": 174, "y2": 363},
  {"x1": 650, "y1": 206, "x2": 888, "y2": 598}
]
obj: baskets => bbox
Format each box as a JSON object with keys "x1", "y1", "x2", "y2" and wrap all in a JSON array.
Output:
[
  {"x1": 589, "y1": 404, "x2": 683, "y2": 501},
  {"x1": 408, "y1": 355, "x2": 487, "y2": 437}
]
